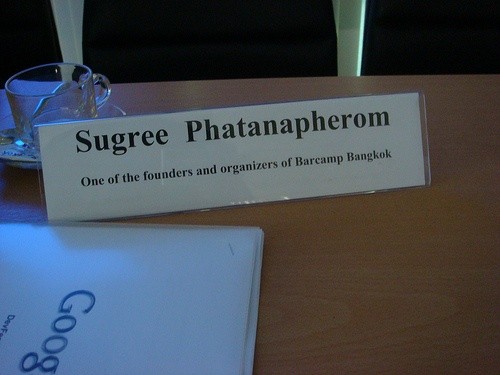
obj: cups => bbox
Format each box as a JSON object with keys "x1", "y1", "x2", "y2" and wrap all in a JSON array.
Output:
[{"x1": 5, "y1": 62, "x2": 111, "y2": 148}]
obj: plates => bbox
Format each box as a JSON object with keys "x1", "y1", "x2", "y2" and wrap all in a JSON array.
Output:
[{"x1": 0, "y1": 104, "x2": 127, "y2": 169}]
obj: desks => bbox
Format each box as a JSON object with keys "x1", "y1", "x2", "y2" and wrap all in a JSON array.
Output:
[{"x1": 1, "y1": 75, "x2": 498, "y2": 375}]
